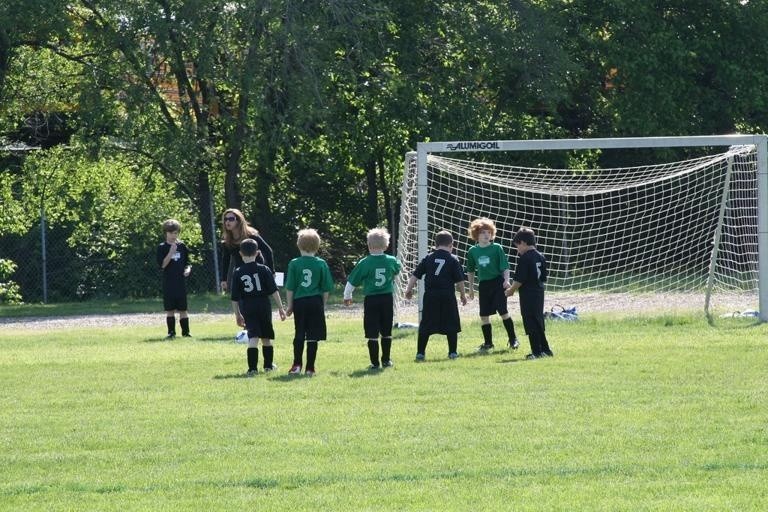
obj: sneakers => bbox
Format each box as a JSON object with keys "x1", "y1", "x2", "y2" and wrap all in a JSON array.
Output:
[
  {"x1": 182, "y1": 333, "x2": 191, "y2": 337},
  {"x1": 247, "y1": 368, "x2": 258, "y2": 375},
  {"x1": 416, "y1": 353, "x2": 424, "y2": 362},
  {"x1": 449, "y1": 352, "x2": 457, "y2": 359},
  {"x1": 236, "y1": 334, "x2": 249, "y2": 343},
  {"x1": 167, "y1": 333, "x2": 176, "y2": 339},
  {"x1": 371, "y1": 362, "x2": 379, "y2": 369},
  {"x1": 382, "y1": 360, "x2": 393, "y2": 367},
  {"x1": 481, "y1": 344, "x2": 493, "y2": 353},
  {"x1": 509, "y1": 339, "x2": 519, "y2": 348},
  {"x1": 526, "y1": 349, "x2": 553, "y2": 360},
  {"x1": 289, "y1": 364, "x2": 302, "y2": 374},
  {"x1": 305, "y1": 368, "x2": 314, "y2": 375},
  {"x1": 264, "y1": 365, "x2": 275, "y2": 373}
]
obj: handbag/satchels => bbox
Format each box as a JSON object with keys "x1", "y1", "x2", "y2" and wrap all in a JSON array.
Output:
[{"x1": 543, "y1": 304, "x2": 577, "y2": 320}]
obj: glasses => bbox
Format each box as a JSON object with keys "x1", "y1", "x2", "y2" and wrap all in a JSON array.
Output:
[{"x1": 224, "y1": 217, "x2": 235, "y2": 221}]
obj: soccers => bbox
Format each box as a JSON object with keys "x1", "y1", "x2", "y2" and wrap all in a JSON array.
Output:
[{"x1": 235, "y1": 330, "x2": 249, "y2": 343}]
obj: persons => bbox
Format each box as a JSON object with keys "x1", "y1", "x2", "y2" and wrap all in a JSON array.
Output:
[
  {"x1": 156, "y1": 220, "x2": 193, "y2": 339},
  {"x1": 503, "y1": 227, "x2": 553, "y2": 359},
  {"x1": 405, "y1": 231, "x2": 467, "y2": 359},
  {"x1": 343, "y1": 227, "x2": 404, "y2": 369},
  {"x1": 286, "y1": 229, "x2": 335, "y2": 376},
  {"x1": 466, "y1": 217, "x2": 520, "y2": 350},
  {"x1": 230, "y1": 238, "x2": 286, "y2": 374},
  {"x1": 220, "y1": 207, "x2": 275, "y2": 342}
]
